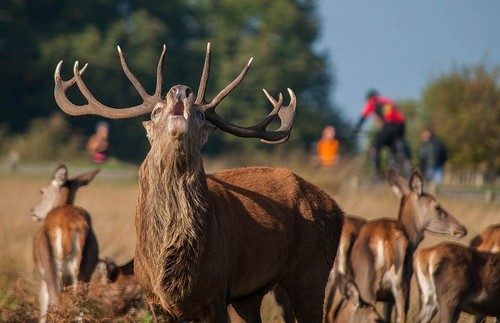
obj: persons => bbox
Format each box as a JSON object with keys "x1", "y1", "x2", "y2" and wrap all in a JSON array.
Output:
[
  {"x1": 416, "y1": 127, "x2": 449, "y2": 185},
  {"x1": 352, "y1": 90, "x2": 413, "y2": 176},
  {"x1": 86, "y1": 122, "x2": 110, "y2": 165},
  {"x1": 317, "y1": 124, "x2": 341, "y2": 174}
]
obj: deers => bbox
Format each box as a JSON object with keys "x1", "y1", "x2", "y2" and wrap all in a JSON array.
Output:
[
  {"x1": 53, "y1": 41, "x2": 345, "y2": 323},
  {"x1": 98, "y1": 255, "x2": 147, "y2": 320},
  {"x1": 30, "y1": 164, "x2": 103, "y2": 323},
  {"x1": 413, "y1": 241, "x2": 500, "y2": 323},
  {"x1": 352, "y1": 169, "x2": 469, "y2": 323},
  {"x1": 467, "y1": 223, "x2": 500, "y2": 251},
  {"x1": 324, "y1": 214, "x2": 370, "y2": 323}
]
